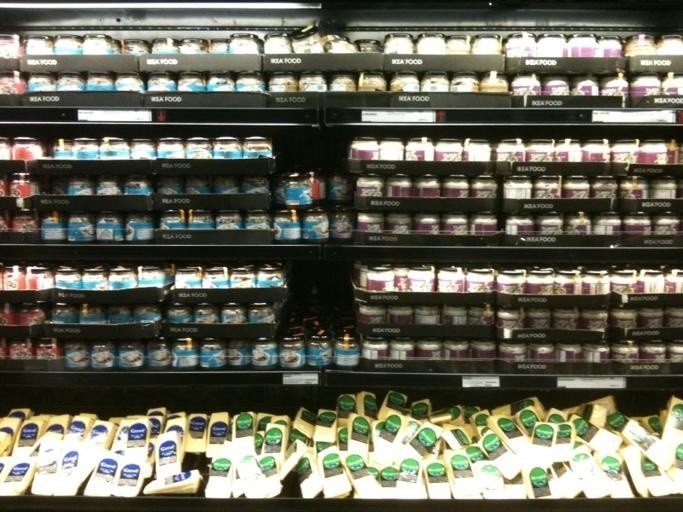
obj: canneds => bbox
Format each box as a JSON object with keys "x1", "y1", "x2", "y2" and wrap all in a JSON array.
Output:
[
  {"x1": 274, "y1": 169, "x2": 354, "y2": 244},
  {"x1": 545, "y1": 75, "x2": 570, "y2": 95},
  {"x1": 407, "y1": 138, "x2": 434, "y2": 161},
  {"x1": 466, "y1": 139, "x2": 492, "y2": 162},
  {"x1": 390, "y1": 71, "x2": 419, "y2": 91},
  {"x1": 527, "y1": 139, "x2": 555, "y2": 162},
  {"x1": 0, "y1": 25, "x2": 382, "y2": 56},
  {"x1": 570, "y1": 33, "x2": 599, "y2": 57},
  {"x1": 0, "y1": 261, "x2": 288, "y2": 292},
  {"x1": 495, "y1": 138, "x2": 526, "y2": 162},
  {"x1": 634, "y1": 74, "x2": 663, "y2": 96},
  {"x1": 1, "y1": 172, "x2": 272, "y2": 198},
  {"x1": 537, "y1": 33, "x2": 567, "y2": 57},
  {"x1": 356, "y1": 211, "x2": 682, "y2": 236},
  {"x1": 0, "y1": 336, "x2": 277, "y2": 372},
  {"x1": 1, "y1": 135, "x2": 273, "y2": 162},
  {"x1": 659, "y1": 34, "x2": 683, "y2": 56},
  {"x1": 416, "y1": 33, "x2": 446, "y2": 54},
  {"x1": 482, "y1": 71, "x2": 509, "y2": 93},
  {"x1": 600, "y1": 35, "x2": 622, "y2": 58},
  {"x1": 436, "y1": 138, "x2": 464, "y2": 162},
  {"x1": 471, "y1": 33, "x2": 500, "y2": 54},
  {"x1": 0, "y1": 71, "x2": 358, "y2": 94},
  {"x1": 451, "y1": 71, "x2": 480, "y2": 92},
  {"x1": 624, "y1": 34, "x2": 657, "y2": 56},
  {"x1": 602, "y1": 75, "x2": 630, "y2": 96},
  {"x1": 383, "y1": 33, "x2": 414, "y2": 55},
  {"x1": 349, "y1": 135, "x2": 379, "y2": 161},
  {"x1": 1, "y1": 301, "x2": 276, "y2": 326},
  {"x1": 356, "y1": 173, "x2": 683, "y2": 198},
  {"x1": 421, "y1": 68, "x2": 450, "y2": 92},
  {"x1": 510, "y1": 71, "x2": 541, "y2": 96},
  {"x1": 502, "y1": 33, "x2": 536, "y2": 57},
  {"x1": 357, "y1": 72, "x2": 387, "y2": 93},
  {"x1": 361, "y1": 340, "x2": 682, "y2": 363},
  {"x1": 557, "y1": 139, "x2": 683, "y2": 164},
  {"x1": 574, "y1": 76, "x2": 600, "y2": 95},
  {"x1": 279, "y1": 298, "x2": 360, "y2": 369},
  {"x1": 665, "y1": 76, "x2": 683, "y2": 97},
  {"x1": 446, "y1": 35, "x2": 468, "y2": 54},
  {"x1": 350, "y1": 263, "x2": 683, "y2": 295},
  {"x1": 380, "y1": 139, "x2": 405, "y2": 160},
  {"x1": 357, "y1": 304, "x2": 682, "y2": 330},
  {"x1": 0, "y1": 209, "x2": 275, "y2": 244}
]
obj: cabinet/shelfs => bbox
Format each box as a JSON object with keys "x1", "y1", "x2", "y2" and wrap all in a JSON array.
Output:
[{"x1": 2, "y1": 0, "x2": 683, "y2": 510}]
